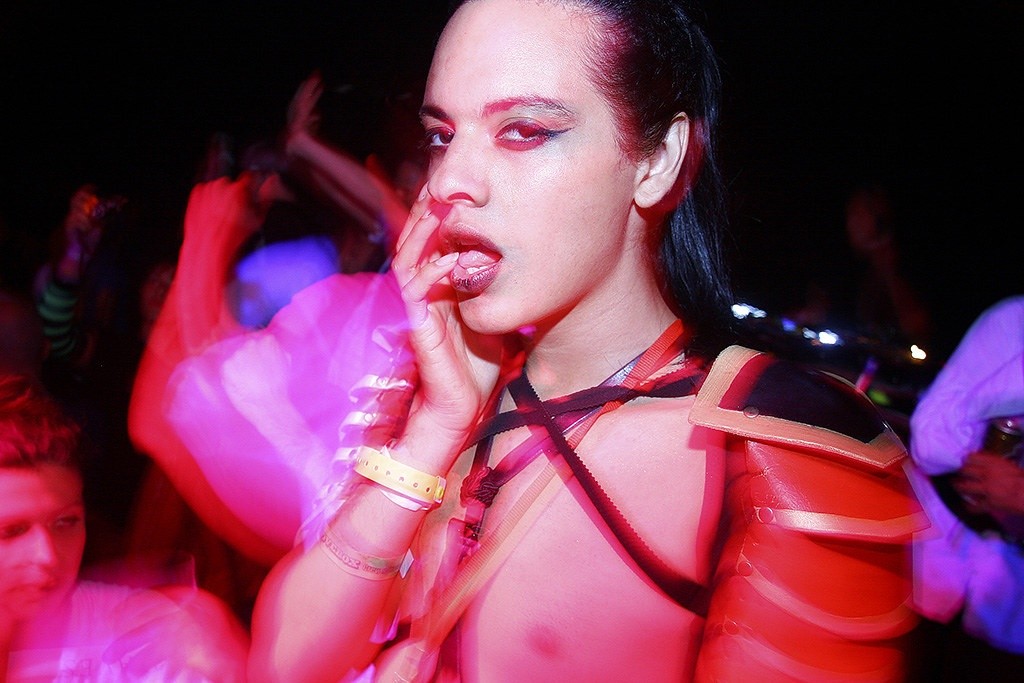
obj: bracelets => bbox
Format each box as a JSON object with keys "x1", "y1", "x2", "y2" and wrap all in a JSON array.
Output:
[
  {"x1": 312, "y1": 521, "x2": 405, "y2": 582},
  {"x1": 353, "y1": 442, "x2": 447, "y2": 514}
]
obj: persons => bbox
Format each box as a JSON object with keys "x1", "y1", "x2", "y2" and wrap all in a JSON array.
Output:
[{"x1": 0, "y1": 0, "x2": 1024, "y2": 683}]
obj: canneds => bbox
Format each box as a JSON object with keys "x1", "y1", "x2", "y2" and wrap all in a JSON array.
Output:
[{"x1": 960, "y1": 417, "x2": 1024, "y2": 506}]
